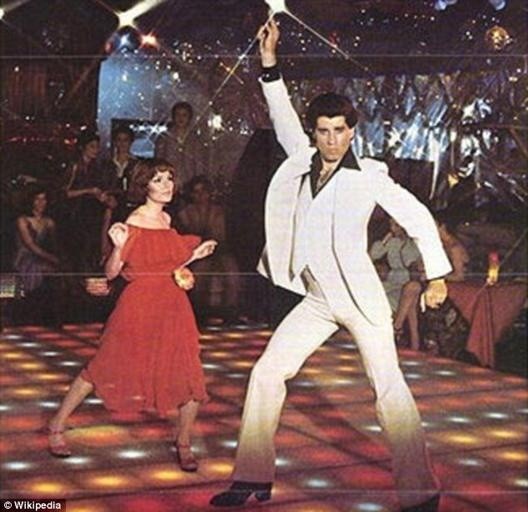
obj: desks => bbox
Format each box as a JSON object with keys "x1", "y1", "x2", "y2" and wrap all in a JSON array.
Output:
[{"x1": 445, "y1": 279, "x2": 527, "y2": 369}]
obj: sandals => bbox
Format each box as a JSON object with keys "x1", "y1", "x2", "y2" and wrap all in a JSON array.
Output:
[{"x1": 393, "y1": 326, "x2": 403, "y2": 336}]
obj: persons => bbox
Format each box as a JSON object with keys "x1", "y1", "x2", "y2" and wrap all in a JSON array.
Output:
[
  {"x1": 44, "y1": 158, "x2": 220, "y2": 472},
  {"x1": 12, "y1": 102, "x2": 471, "y2": 353},
  {"x1": 208, "y1": 13, "x2": 455, "y2": 510}
]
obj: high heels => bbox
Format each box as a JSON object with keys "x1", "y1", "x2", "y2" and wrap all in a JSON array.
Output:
[
  {"x1": 44, "y1": 420, "x2": 71, "y2": 458},
  {"x1": 174, "y1": 441, "x2": 197, "y2": 472}
]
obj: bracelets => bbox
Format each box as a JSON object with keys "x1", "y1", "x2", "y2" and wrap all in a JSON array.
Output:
[{"x1": 260, "y1": 65, "x2": 282, "y2": 83}]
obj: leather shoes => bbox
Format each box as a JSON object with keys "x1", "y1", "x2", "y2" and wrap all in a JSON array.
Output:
[
  {"x1": 209, "y1": 482, "x2": 271, "y2": 507},
  {"x1": 401, "y1": 491, "x2": 441, "y2": 511}
]
